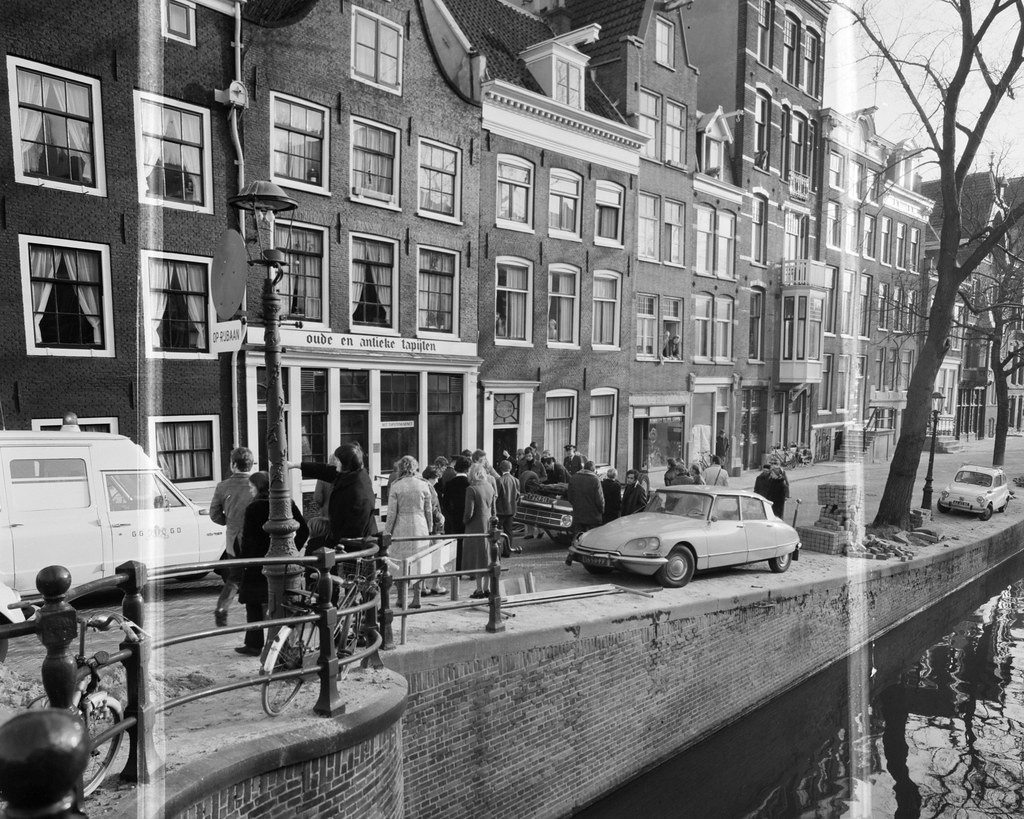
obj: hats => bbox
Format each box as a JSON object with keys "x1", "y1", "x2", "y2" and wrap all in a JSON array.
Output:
[
  {"x1": 719, "y1": 429, "x2": 726, "y2": 434},
  {"x1": 540, "y1": 450, "x2": 556, "y2": 461},
  {"x1": 763, "y1": 464, "x2": 771, "y2": 469},
  {"x1": 563, "y1": 445, "x2": 577, "y2": 452}
]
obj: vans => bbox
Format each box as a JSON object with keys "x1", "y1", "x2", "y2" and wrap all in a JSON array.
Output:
[{"x1": 0, "y1": 430, "x2": 228, "y2": 631}]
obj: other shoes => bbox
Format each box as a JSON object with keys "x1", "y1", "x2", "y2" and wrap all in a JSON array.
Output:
[
  {"x1": 396, "y1": 599, "x2": 403, "y2": 607},
  {"x1": 408, "y1": 597, "x2": 421, "y2": 609},
  {"x1": 432, "y1": 586, "x2": 447, "y2": 595},
  {"x1": 421, "y1": 588, "x2": 431, "y2": 596},
  {"x1": 235, "y1": 644, "x2": 261, "y2": 657},
  {"x1": 213, "y1": 606, "x2": 228, "y2": 627}
]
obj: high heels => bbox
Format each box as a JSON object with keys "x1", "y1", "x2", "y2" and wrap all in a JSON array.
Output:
[
  {"x1": 484, "y1": 590, "x2": 491, "y2": 598},
  {"x1": 470, "y1": 589, "x2": 484, "y2": 599}
]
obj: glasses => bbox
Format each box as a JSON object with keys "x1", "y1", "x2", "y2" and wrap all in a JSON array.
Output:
[
  {"x1": 541, "y1": 458, "x2": 554, "y2": 465},
  {"x1": 566, "y1": 448, "x2": 571, "y2": 452},
  {"x1": 770, "y1": 462, "x2": 777, "y2": 466}
]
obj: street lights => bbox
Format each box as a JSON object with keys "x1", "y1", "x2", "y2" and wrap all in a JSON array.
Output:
[
  {"x1": 228, "y1": 180, "x2": 307, "y2": 679},
  {"x1": 921, "y1": 389, "x2": 948, "y2": 518}
]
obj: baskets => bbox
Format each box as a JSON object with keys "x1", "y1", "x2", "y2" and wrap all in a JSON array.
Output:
[{"x1": 338, "y1": 562, "x2": 379, "y2": 593}]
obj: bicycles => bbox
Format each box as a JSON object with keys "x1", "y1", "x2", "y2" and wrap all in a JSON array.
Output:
[
  {"x1": 765, "y1": 444, "x2": 800, "y2": 471},
  {"x1": 698, "y1": 450, "x2": 725, "y2": 472},
  {"x1": 258, "y1": 536, "x2": 403, "y2": 719},
  {"x1": 5, "y1": 599, "x2": 154, "y2": 802},
  {"x1": 787, "y1": 441, "x2": 813, "y2": 468}
]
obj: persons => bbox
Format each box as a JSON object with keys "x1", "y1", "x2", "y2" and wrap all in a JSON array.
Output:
[
  {"x1": 717, "y1": 430, "x2": 728, "y2": 468},
  {"x1": 664, "y1": 455, "x2": 730, "y2": 486},
  {"x1": 754, "y1": 464, "x2": 789, "y2": 522},
  {"x1": 209, "y1": 442, "x2": 379, "y2": 656},
  {"x1": 548, "y1": 319, "x2": 558, "y2": 341},
  {"x1": 664, "y1": 330, "x2": 681, "y2": 360},
  {"x1": 385, "y1": 450, "x2": 521, "y2": 608},
  {"x1": 503, "y1": 440, "x2": 648, "y2": 570},
  {"x1": 496, "y1": 312, "x2": 505, "y2": 336}
]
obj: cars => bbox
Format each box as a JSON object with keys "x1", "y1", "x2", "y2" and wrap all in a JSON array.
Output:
[
  {"x1": 570, "y1": 485, "x2": 802, "y2": 588},
  {"x1": 936, "y1": 463, "x2": 1014, "y2": 521}
]
